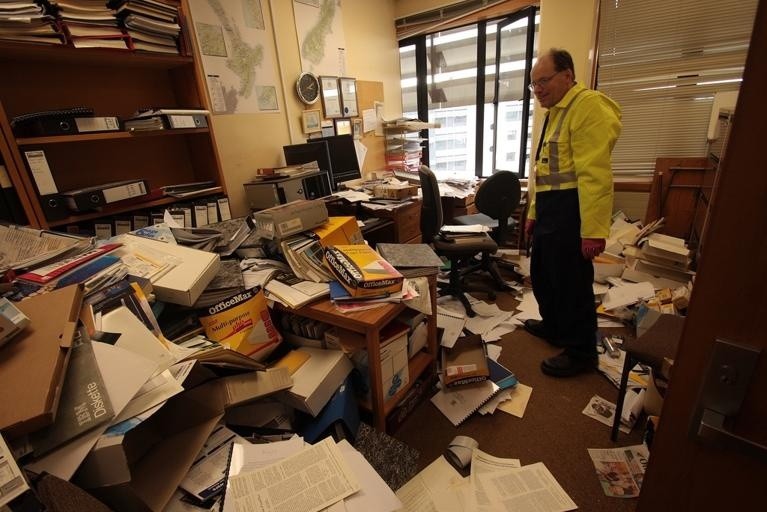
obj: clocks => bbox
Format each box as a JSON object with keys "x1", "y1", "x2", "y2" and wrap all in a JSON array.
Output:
[{"x1": 296, "y1": 71, "x2": 320, "y2": 105}]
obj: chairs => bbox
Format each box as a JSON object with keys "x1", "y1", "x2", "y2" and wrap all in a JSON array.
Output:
[
  {"x1": 418, "y1": 165, "x2": 498, "y2": 318},
  {"x1": 451, "y1": 171, "x2": 525, "y2": 291}
]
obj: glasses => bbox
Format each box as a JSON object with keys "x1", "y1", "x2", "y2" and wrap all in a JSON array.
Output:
[{"x1": 527, "y1": 71, "x2": 563, "y2": 91}]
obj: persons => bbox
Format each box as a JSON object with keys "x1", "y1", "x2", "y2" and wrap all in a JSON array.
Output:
[{"x1": 525, "y1": 48, "x2": 623, "y2": 377}]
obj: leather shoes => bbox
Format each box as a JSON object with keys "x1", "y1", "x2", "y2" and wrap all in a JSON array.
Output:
[
  {"x1": 523, "y1": 319, "x2": 561, "y2": 343},
  {"x1": 541, "y1": 350, "x2": 597, "y2": 379}
]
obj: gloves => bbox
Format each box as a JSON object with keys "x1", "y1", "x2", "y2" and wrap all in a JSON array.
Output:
[
  {"x1": 581, "y1": 238, "x2": 606, "y2": 259},
  {"x1": 524, "y1": 220, "x2": 535, "y2": 240}
]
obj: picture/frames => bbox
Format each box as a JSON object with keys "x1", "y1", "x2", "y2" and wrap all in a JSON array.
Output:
[
  {"x1": 318, "y1": 75, "x2": 343, "y2": 119},
  {"x1": 302, "y1": 109, "x2": 322, "y2": 134},
  {"x1": 339, "y1": 77, "x2": 359, "y2": 119},
  {"x1": 333, "y1": 118, "x2": 354, "y2": 136}
]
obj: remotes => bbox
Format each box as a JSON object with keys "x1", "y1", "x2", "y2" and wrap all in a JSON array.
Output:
[{"x1": 263, "y1": 175, "x2": 289, "y2": 181}]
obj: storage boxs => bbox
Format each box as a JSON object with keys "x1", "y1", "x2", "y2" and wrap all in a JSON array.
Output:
[
  {"x1": 310, "y1": 216, "x2": 365, "y2": 252},
  {"x1": 278, "y1": 315, "x2": 324, "y2": 347},
  {"x1": 324, "y1": 323, "x2": 410, "y2": 406}
]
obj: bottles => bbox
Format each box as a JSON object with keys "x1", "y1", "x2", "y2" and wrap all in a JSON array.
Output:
[{"x1": 602, "y1": 333, "x2": 621, "y2": 358}]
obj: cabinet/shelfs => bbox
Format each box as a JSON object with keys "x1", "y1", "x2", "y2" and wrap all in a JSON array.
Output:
[
  {"x1": 383, "y1": 122, "x2": 423, "y2": 173},
  {"x1": 0, "y1": 0, "x2": 232, "y2": 230}
]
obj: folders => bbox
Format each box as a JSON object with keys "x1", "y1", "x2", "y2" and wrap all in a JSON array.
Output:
[
  {"x1": 17, "y1": 116, "x2": 123, "y2": 135},
  {"x1": 330, "y1": 280, "x2": 390, "y2": 301},
  {"x1": 376, "y1": 243, "x2": 446, "y2": 278},
  {"x1": 21, "y1": 148, "x2": 69, "y2": 222},
  {"x1": 122, "y1": 115, "x2": 210, "y2": 132},
  {"x1": 0, "y1": 21, "x2": 69, "y2": 46},
  {"x1": 64, "y1": 178, "x2": 152, "y2": 213},
  {"x1": 66, "y1": 198, "x2": 232, "y2": 239}
]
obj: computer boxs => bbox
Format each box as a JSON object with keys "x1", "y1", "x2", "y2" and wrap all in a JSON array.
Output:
[{"x1": 243, "y1": 170, "x2": 333, "y2": 209}]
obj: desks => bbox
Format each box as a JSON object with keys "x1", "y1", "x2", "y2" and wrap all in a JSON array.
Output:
[
  {"x1": 271, "y1": 273, "x2": 439, "y2": 433},
  {"x1": 360, "y1": 199, "x2": 422, "y2": 251}
]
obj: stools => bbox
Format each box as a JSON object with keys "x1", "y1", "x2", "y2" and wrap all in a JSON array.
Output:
[{"x1": 611, "y1": 314, "x2": 685, "y2": 441}]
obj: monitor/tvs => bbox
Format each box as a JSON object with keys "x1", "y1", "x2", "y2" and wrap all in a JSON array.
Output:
[
  {"x1": 306, "y1": 134, "x2": 361, "y2": 193},
  {"x1": 282, "y1": 140, "x2": 336, "y2": 190}
]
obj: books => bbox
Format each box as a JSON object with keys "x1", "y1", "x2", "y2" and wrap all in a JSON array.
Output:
[
  {"x1": 0, "y1": 108, "x2": 695, "y2": 512},
  {"x1": 0, "y1": 0, "x2": 181, "y2": 54}
]
